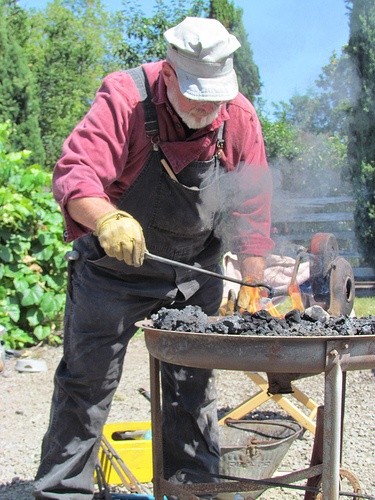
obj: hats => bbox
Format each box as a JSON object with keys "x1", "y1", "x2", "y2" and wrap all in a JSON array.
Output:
[{"x1": 163, "y1": 16, "x2": 241, "y2": 101}]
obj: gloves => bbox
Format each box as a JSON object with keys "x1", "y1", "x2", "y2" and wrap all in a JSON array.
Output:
[
  {"x1": 219, "y1": 278, "x2": 264, "y2": 316},
  {"x1": 94, "y1": 211, "x2": 150, "y2": 267}
]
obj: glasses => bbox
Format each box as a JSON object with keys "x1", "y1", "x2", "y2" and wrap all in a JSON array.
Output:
[{"x1": 167, "y1": 62, "x2": 227, "y2": 105}]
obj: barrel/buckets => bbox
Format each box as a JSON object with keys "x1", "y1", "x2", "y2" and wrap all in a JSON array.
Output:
[{"x1": 215, "y1": 417, "x2": 303, "y2": 499}]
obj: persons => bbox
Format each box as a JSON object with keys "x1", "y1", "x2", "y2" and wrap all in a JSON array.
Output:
[{"x1": 30, "y1": 17, "x2": 275, "y2": 500}]
